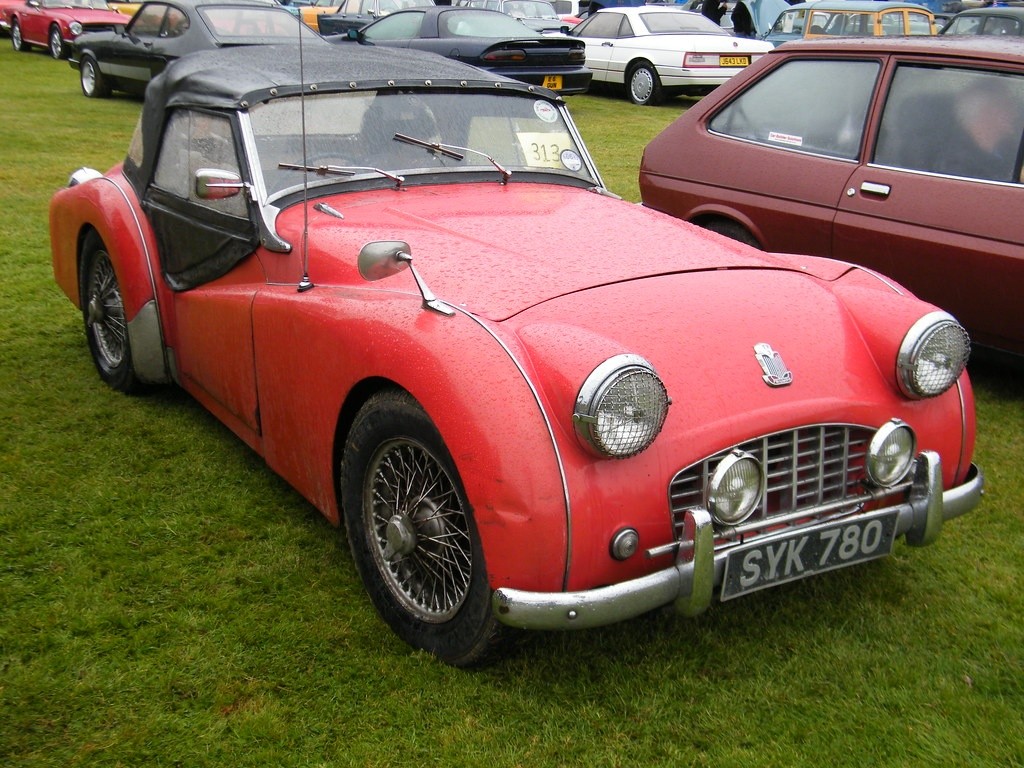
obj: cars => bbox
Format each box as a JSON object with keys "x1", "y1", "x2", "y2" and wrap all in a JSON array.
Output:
[
  {"x1": 636, "y1": 35, "x2": 1024, "y2": 356},
  {"x1": 50, "y1": 9, "x2": 986, "y2": 674},
  {"x1": 0, "y1": 0, "x2": 1024, "y2": 105}
]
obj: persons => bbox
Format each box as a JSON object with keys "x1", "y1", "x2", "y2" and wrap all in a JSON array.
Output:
[
  {"x1": 702, "y1": 0, "x2": 727, "y2": 25},
  {"x1": 731, "y1": 0, "x2": 752, "y2": 36},
  {"x1": 980, "y1": 0, "x2": 1008, "y2": 7}
]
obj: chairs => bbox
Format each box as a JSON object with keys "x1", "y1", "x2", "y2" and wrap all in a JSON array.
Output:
[
  {"x1": 810, "y1": 15, "x2": 847, "y2": 36},
  {"x1": 891, "y1": 87, "x2": 991, "y2": 181},
  {"x1": 360, "y1": 89, "x2": 442, "y2": 169}
]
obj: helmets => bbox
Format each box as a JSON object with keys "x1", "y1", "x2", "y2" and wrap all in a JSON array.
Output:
[{"x1": 362, "y1": 90, "x2": 441, "y2": 173}]
